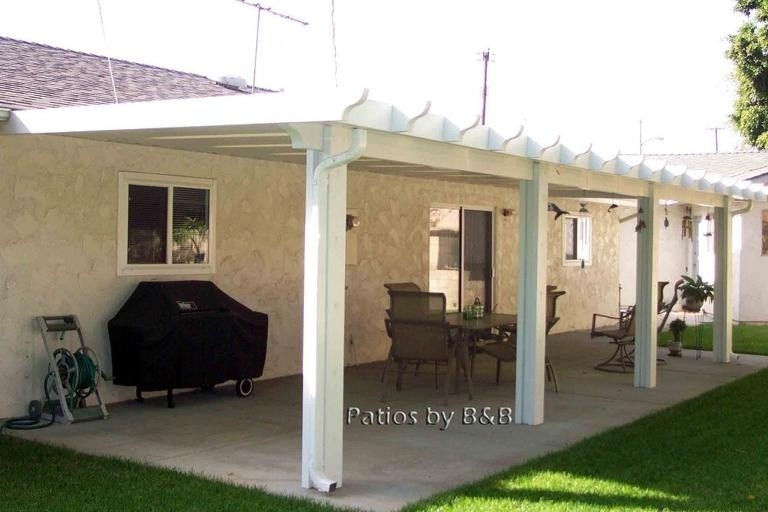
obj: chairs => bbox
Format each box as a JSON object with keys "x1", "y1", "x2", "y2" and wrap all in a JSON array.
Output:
[
  {"x1": 378, "y1": 280, "x2": 566, "y2": 401},
  {"x1": 590, "y1": 278, "x2": 684, "y2": 376}
]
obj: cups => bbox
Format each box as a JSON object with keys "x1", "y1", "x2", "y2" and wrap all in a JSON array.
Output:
[{"x1": 462, "y1": 304, "x2": 474, "y2": 320}]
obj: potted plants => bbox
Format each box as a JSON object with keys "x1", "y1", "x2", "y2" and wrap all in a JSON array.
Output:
[{"x1": 679, "y1": 273, "x2": 715, "y2": 314}]
